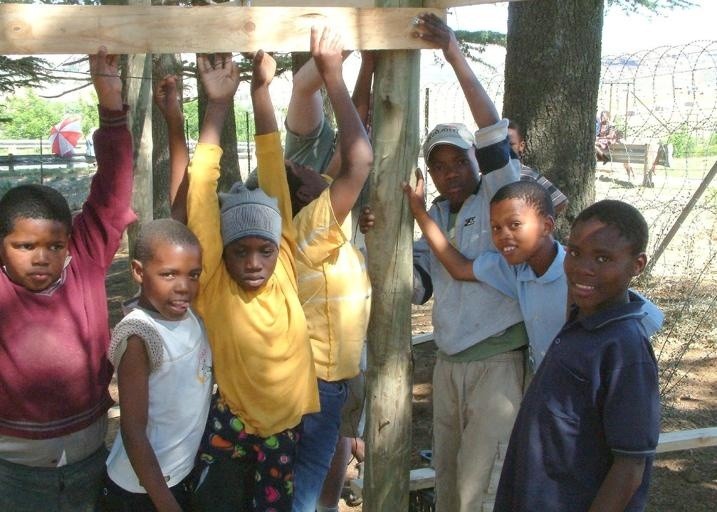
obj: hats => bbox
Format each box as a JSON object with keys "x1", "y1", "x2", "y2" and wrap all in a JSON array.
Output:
[
  {"x1": 217, "y1": 182, "x2": 282, "y2": 250},
  {"x1": 422, "y1": 122, "x2": 475, "y2": 165}
]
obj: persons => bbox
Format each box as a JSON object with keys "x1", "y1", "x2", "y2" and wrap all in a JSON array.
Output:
[
  {"x1": 284, "y1": 49, "x2": 376, "y2": 506},
  {"x1": 506, "y1": 117, "x2": 568, "y2": 222},
  {"x1": 491, "y1": 199, "x2": 661, "y2": 512},
  {"x1": 108, "y1": 72, "x2": 216, "y2": 512},
  {"x1": 186, "y1": 52, "x2": 320, "y2": 512},
  {"x1": 401, "y1": 169, "x2": 568, "y2": 379},
  {"x1": 242, "y1": 24, "x2": 375, "y2": 512},
  {"x1": 0, "y1": 44, "x2": 135, "y2": 512},
  {"x1": 593, "y1": 110, "x2": 616, "y2": 162},
  {"x1": 358, "y1": 12, "x2": 530, "y2": 512}
]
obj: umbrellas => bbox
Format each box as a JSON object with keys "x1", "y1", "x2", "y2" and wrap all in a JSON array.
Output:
[{"x1": 49, "y1": 116, "x2": 84, "y2": 157}]
